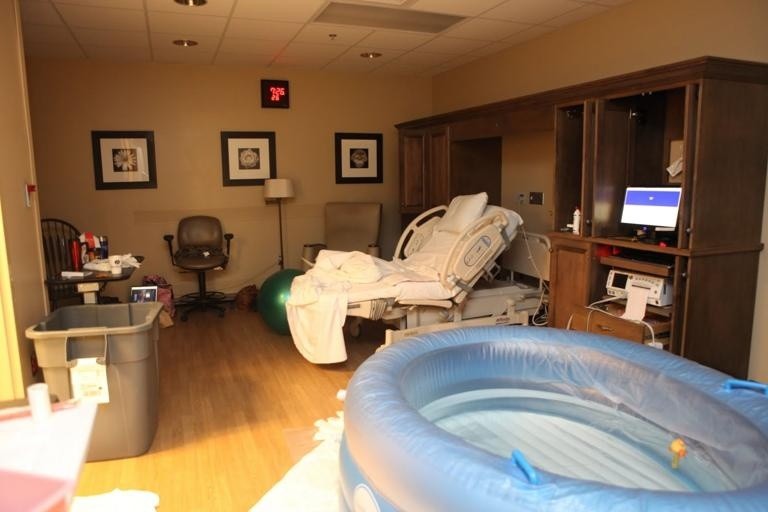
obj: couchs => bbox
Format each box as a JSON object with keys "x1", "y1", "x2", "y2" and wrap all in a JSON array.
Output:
[{"x1": 300, "y1": 200, "x2": 382, "y2": 272}]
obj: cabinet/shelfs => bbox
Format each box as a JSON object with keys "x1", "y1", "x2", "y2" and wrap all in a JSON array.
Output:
[{"x1": 393, "y1": 54, "x2": 768, "y2": 379}]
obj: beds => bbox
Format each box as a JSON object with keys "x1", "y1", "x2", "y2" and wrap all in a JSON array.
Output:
[{"x1": 286, "y1": 192, "x2": 524, "y2": 365}]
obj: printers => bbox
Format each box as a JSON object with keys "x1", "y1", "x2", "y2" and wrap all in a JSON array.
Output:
[{"x1": 605, "y1": 270, "x2": 673, "y2": 307}]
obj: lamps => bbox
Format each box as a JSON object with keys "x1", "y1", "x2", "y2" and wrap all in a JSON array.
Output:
[{"x1": 263, "y1": 178, "x2": 297, "y2": 271}]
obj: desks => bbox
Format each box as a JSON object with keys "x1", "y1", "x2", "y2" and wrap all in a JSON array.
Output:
[{"x1": 44, "y1": 255, "x2": 145, "y2": 306}]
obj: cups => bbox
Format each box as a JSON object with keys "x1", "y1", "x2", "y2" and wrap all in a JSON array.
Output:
[{"x1": 108, "y1": 255, "x2": 123, "y2": 275}]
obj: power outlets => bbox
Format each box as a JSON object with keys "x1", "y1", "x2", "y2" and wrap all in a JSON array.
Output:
[{"x1": 517, "y1": 191, "x2": 528, "y2": 206}]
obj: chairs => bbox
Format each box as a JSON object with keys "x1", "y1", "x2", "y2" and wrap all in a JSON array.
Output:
[
  {"x1": 163, "y1": 216, "x2": 233, "y2": 321},
  {"x1": 41, "y1": 216, "x2": 105, "y2": 306}
]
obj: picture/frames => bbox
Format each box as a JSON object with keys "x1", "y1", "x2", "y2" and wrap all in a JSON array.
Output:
[
  {"x1": 334, "y1": 131, "x2": 384, "y2": 185},
  {"x1": 91, "y1": 130, "x2": 156, "y2": 190},
  {"x1": 221, "y1": 129, "x2": 277, "y2": 187}
]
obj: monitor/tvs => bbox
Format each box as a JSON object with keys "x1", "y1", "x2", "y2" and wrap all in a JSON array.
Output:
[{"x1": 619, "y1": 185, "x2": 682, "y2": 245}]
